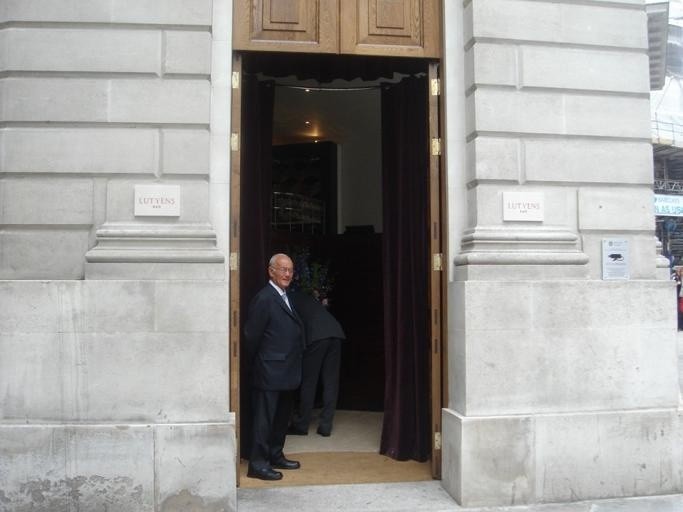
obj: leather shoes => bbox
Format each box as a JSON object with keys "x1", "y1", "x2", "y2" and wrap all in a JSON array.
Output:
[
  {"x1": 246, "y1": 465, "x2": 284, "y2": 481},
  {"x1": 285, "y1": 426, "x2": 308, "y2": 435},
  {"x1": 317, "y1": 426, "x2": 331, "y2": 436},
  {"x1": 270, "y1": 456, "x2": 301, "y2": 470}
]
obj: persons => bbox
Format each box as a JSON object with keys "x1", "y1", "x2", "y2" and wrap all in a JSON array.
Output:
[{"x1": 243, "y1": 252, "x2": 346, "y2": 480}]
